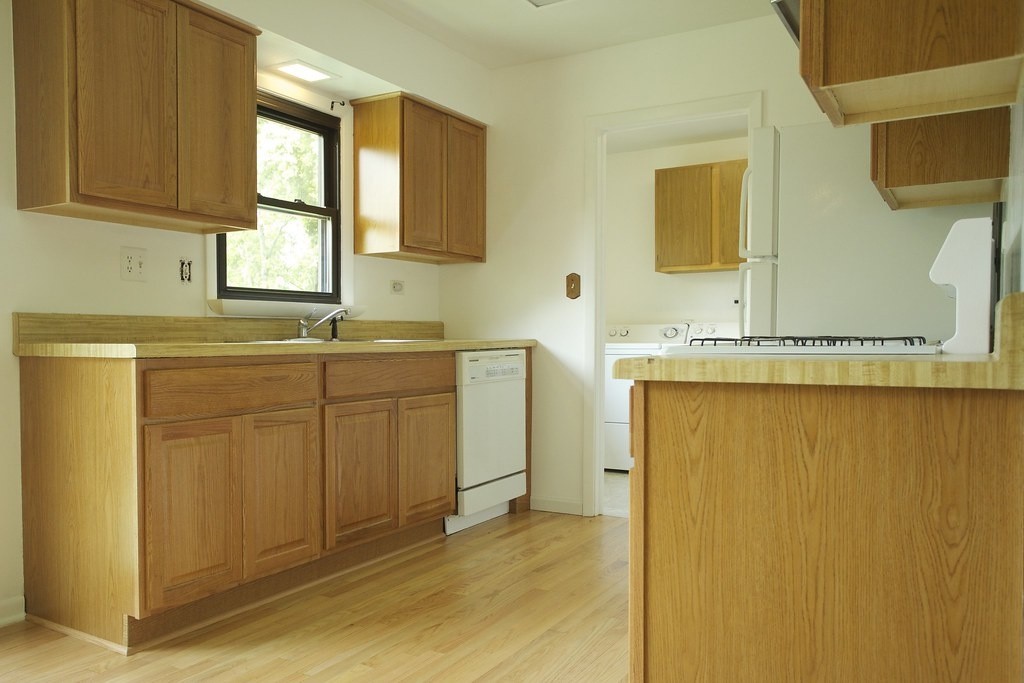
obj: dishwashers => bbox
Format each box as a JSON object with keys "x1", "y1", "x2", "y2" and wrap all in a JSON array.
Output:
[{"x1": 455, "y1": 349, "x2": 527, "y2": 515}]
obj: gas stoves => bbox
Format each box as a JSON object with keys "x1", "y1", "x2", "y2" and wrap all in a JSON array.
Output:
[{"x1": 663, "y1": 335, "x2": 941, "y2": 353}]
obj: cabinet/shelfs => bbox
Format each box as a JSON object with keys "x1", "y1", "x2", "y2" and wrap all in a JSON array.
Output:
[
  {"x1": 349, "y1": 91, "x2": 488, "y2": 265},
  {"x1": 323, "y1": 351, "x2": 458, "y2": 581},
  {"x1": 629, "y1": 380, "x2": 1024, "y2": 683},
  {"x1": 18, "y1": 353, "x2": 325, "y2": 658},
  {"x1": 799, "y1": 0, "x2": 1024, "y2": 127},
  {"x1": 655, "y1": 158, "x2": 747, "y2": 274},
  {"x1": 12, "y1": 0, "x2": 263, "y2": 234},
  {"x1": 870, "y1": 106, "x2": 1010, "y2": 211}
]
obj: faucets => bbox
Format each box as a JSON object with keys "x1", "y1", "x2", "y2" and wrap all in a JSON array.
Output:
[{"x1": 298, "y1": 308, "x2": 351, "y2": 339}]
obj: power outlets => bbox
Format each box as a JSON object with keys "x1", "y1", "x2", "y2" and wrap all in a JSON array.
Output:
[
  {"x1": 120, "y1": 246, "x2": 147, "y2": 282},
  {"x1": 390, "y1": 280, "x2": 405, "y2": 295},
  {"x1": 180, "y1": 260, "x2": 193, "y2": 284},
  {"x1": 566, "y1": 273, "x2": 580, "y2": 299}
]
